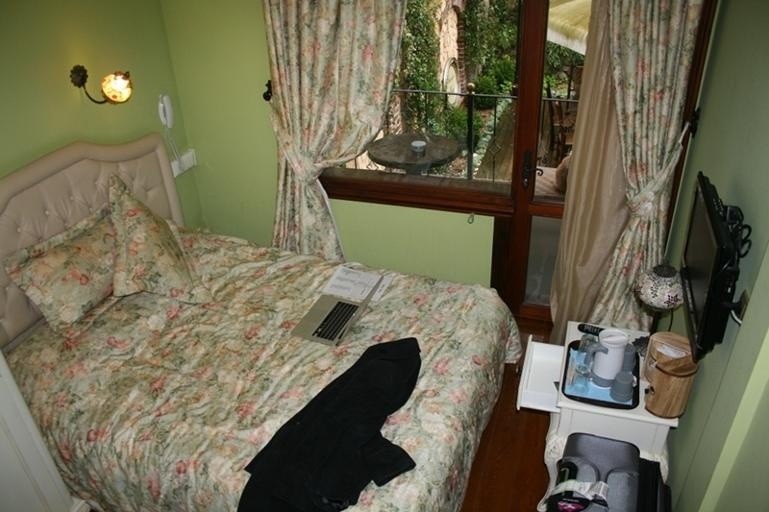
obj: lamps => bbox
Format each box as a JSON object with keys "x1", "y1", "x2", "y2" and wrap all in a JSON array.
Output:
[
  {"x1": 634, "y1": 263, "x2": 685, "y2": 358},
  {"x1": 70, "y1": 63, "x2": 133, "y2": 105}
]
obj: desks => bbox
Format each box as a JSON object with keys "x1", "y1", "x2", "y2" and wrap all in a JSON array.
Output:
[
  {"x1": 368, "y1": 131, "x2": 462, "y2": 177},
  {"x1": 516, "y1": 320, "x2": 680, "y2": 511}
]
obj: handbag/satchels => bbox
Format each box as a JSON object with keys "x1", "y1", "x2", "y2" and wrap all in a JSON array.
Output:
[{"x1": 546, "y1": 431, "x2": 641, "y2": 512}]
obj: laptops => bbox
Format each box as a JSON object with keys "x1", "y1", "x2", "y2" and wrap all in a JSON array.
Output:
[{"x1": 290, "y1": 275, "x2": 384, "y2": 347}]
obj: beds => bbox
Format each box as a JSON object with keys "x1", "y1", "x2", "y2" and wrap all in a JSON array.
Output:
[{"x1": 0, "y1": 130, "x2": 512, "y2": 511}]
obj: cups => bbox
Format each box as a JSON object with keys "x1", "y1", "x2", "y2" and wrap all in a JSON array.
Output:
[
  {"x1": 606, "y1": 344, "x2": 636, "y2": 402},
  {"x1": 570, "y1": 333, "x2": 595, "y2": 394},
  {"x1": 409, "y1": 139, "x2": 426, "y2": 157}
]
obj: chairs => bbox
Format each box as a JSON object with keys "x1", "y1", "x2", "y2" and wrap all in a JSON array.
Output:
[{"x1": 545, "y1": 80, "x2": 576, "y2": 168}]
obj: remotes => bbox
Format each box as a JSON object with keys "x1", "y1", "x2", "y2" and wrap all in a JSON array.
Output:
[{"x1": 578, "y1": 324, "x2": 605, "y2": 335}]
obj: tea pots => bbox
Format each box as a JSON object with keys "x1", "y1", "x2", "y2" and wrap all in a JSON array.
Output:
[{"x1": 586, "y1": 327, "x2": 636, "y2": 387}]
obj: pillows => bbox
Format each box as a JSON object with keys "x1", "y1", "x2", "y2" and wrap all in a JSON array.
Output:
[{"x1": 2, "y1": 172, "x2": 213, "y2": 334}]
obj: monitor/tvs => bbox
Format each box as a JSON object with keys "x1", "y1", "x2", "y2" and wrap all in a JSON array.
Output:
[{"x1": 679, "y1": 171, "x2": 740, "y2": 364}]
